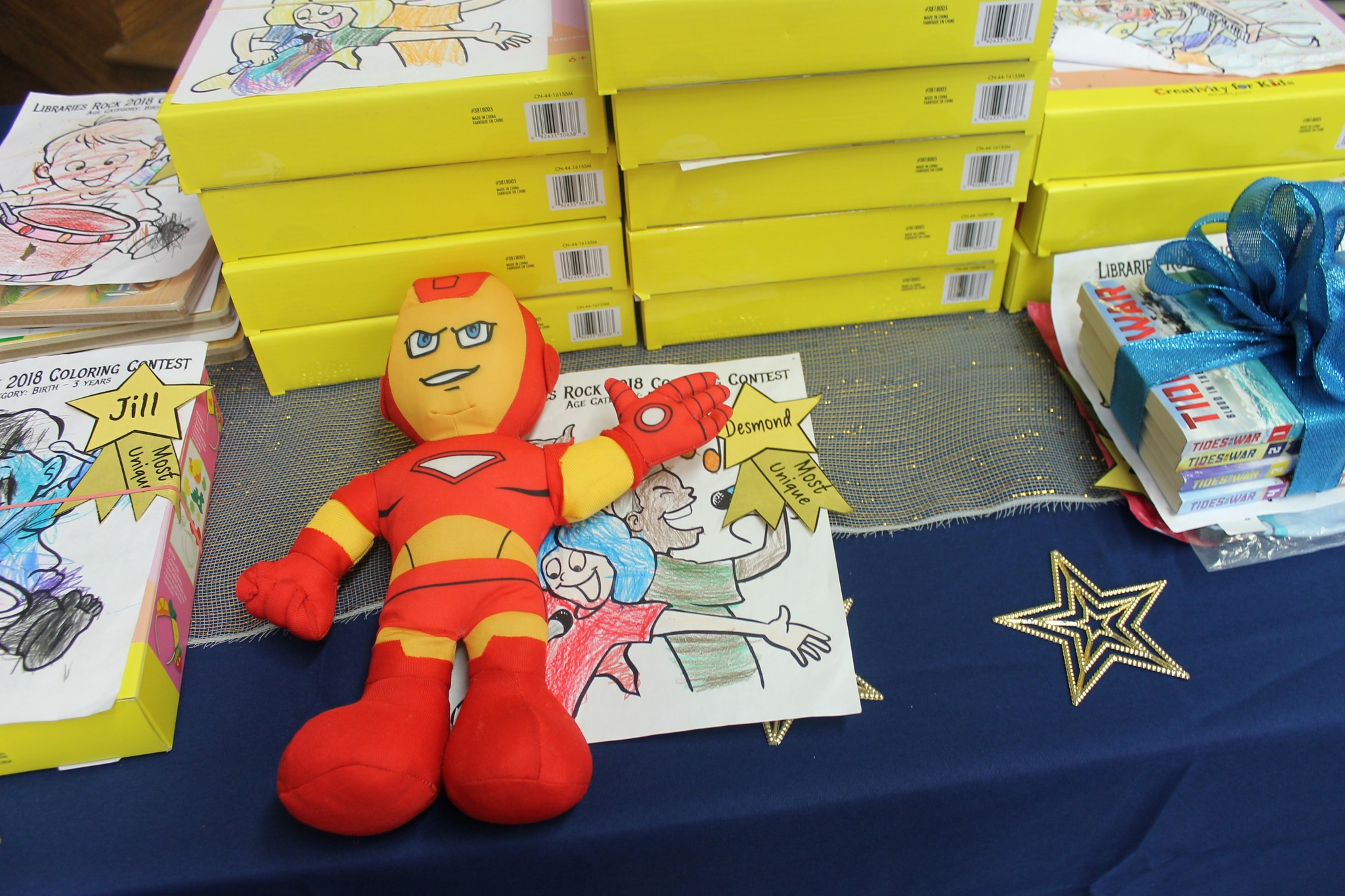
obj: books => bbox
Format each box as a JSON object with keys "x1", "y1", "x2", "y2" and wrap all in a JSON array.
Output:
[
  {"x1": 1078, "y1": 264, "x2": 1345, "y2": 515},
  {"x1": 0, "y1": 232, "x2": 251, "y2": 369}
]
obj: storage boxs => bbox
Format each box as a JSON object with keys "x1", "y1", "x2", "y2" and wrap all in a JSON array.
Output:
[
  {"x1": 196, "y1": 142, "x2": 625, "y2": 264},
  {"x1": 1002, "y1": 229, "x2": 1059, "y2": 317},
  {"x1": 156, "y1": 1, "x2": 612, "y2": 199},
  {"x1": 1, "y1": 363, "x2": 225, "y2": 780},
  {"x1": 611, "y1": 49, "x2": 1052, "y2": 170},
  {"x1": 1032, "y1": 1, "x2": 1345, "y2": 187},
  {"x1": 619, "y1": 133, "x2": 1035, "y2": 232},
  {"x1": 581, "y1": 1, "x2": 1057, "y2": 96},
  {"x1": 1014, "y1": 158, "x2": 1345, "y2": 262},
  {"x1": 246, "y1": 288, "x2": 639, "y2": 396},
  {"x1": 624, "y1": 199, "x2": 1021, "y2": 303},
  {"x1": 636, "y1": 259, "x2": 1010, "y2": 351},
  {"x1": 220, "y1": 217, "x2": 630, "y2": 338}
]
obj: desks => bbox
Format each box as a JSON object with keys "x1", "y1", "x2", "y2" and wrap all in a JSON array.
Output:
[{"x1": 0, "y1": 300, "x2": 1342, "y2": 896}]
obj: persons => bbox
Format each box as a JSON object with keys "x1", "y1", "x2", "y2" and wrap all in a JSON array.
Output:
[{"x1": 234, "y1": 269, "x2": 736, "y2": 837}]
